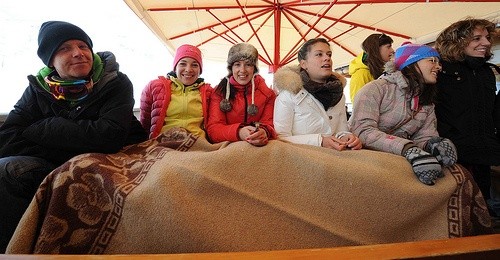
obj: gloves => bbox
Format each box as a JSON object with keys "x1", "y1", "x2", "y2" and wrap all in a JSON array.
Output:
[
  {"x1": 404, "y1": 146, "x2": 445, "y2": 186},
  {"x1": 422, "y1": 136, "x2": 458, "y2": 167}
]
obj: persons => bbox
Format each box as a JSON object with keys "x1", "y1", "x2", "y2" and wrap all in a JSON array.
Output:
[
  {"x1": 419, "y1": 16, "x2": 499, "y2": 260},
  {"x1": 206, "y1": 43, "x2": 277, "y2": 147},
  {"x1": 347, "y1": 44, "x2": 458, "y2": 186},
  {"x1": 273, "y1": 38, "x2": 362, "y2": 152},
  {"x1": 349, "y1": 33, "x2": 394, "y2": 103},
  {"x1": 141, "y1": 45, "x2": 214, "y2": 143},
  {"x1": 0, "y1": 20, "x2": 145, "y2": 254}
]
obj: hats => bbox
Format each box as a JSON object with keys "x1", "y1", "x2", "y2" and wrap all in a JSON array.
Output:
[
  {"x1": 394, "y1": 44, "x2": 441, "y2": 71},
  {"x1": 173, "y1": 44, "x2": 203, "y2": 75},
  {"x1": 36, "y1": 21, "x2": 94, "y2": 70},
  {"x1": 226, "y1": 43, "x2": 259, "y2": 74}
]
